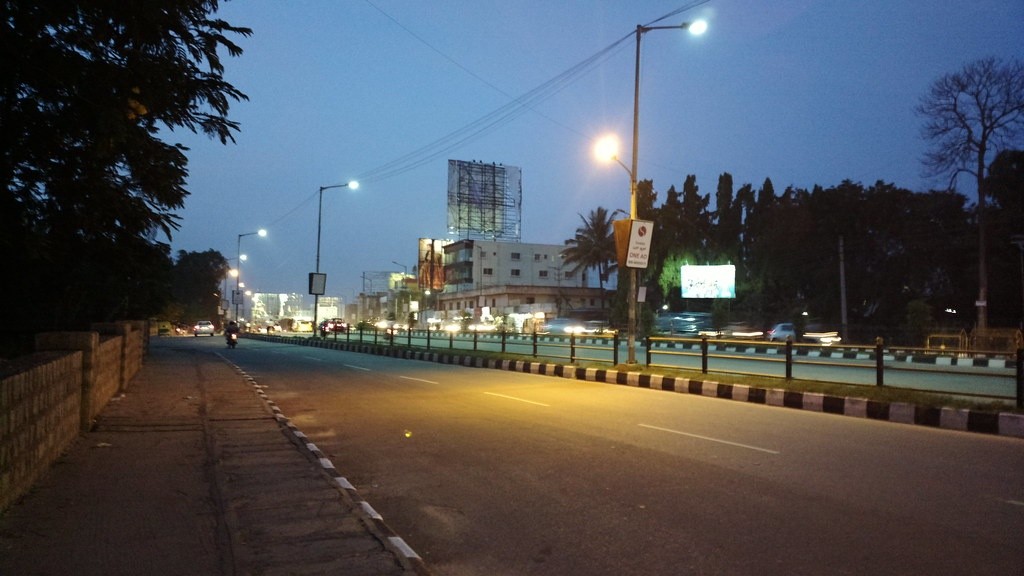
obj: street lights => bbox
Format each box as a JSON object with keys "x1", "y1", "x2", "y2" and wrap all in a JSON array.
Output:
[
  {"x1": 235, "y1": 229, "x2": 266, "y2": 323},
  {"x1": 312, "y1": 181, "x2": 361, "y2": 338},
  {"x1": 590, "y1": 19, "x2": 708, "y2": 363},
  {"x1": 392, "y1": 261, "x2": 408, "y2": 277},
  {"x1": 224, "y1": 253, "x2": 249, "y2": 300}
]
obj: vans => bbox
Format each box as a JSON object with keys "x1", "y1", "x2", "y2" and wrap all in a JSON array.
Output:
[{"x1": 657, "y1": 312, "x2": 714, "y2": 335}]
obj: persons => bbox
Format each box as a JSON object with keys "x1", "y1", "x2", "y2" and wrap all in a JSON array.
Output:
[{"x1": 225, "y1": 320, "x2": 239, "y2": 346}]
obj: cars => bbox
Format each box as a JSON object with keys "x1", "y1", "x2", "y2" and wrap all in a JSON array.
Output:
[
  {"x1": 319, "y1": 317, "x2": 356, "y2": 335},
  {"x1": 356, "y1": 317, "x2": 591, "y2": 338},
  {"x1": 193, "y1": 320, "x2": 215, "y2": 336},
  {"x1": 770, "y1": 322, "x2": 796, "y2": 341}
]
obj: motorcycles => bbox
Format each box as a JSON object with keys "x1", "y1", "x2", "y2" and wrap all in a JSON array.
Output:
[{"x1": 223, "y1": 326, "x2": 239, "y2": 349}]
